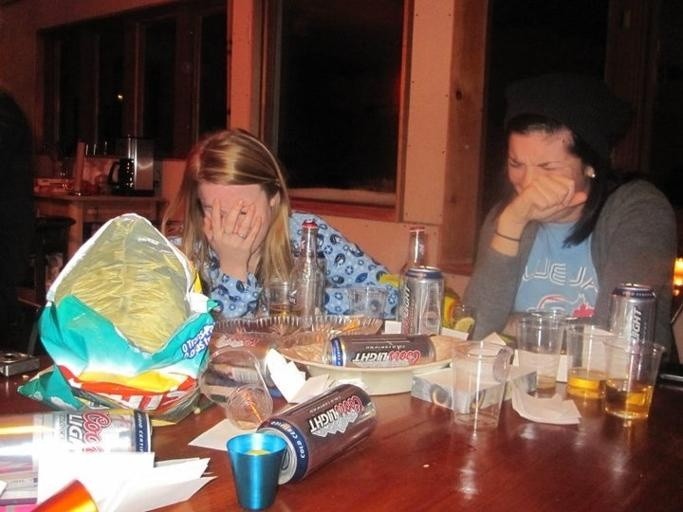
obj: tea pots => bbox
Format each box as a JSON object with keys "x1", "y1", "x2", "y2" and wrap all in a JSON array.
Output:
[{"x1": 107, "y1": 159, "x2": 134, "y2": 194}]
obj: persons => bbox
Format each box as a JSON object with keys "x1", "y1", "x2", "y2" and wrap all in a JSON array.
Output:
[
  {"x1": 446, "y1": 73, "x2": 677, "y2": 386},
  {"x1": 158, "y1": 129, "x2": 408, "y2": 326}
]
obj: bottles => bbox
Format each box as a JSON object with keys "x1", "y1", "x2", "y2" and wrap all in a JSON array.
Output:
[
  {"x1": 289, "y1": 219, "x2": 325, "y2": 318},
  {"x1": 398, "y1": 225, "x2": 427, "y2": 332}
]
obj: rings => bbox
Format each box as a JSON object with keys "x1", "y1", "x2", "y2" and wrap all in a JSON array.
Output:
[{"x1": 237, "y1": 231, "x2": 246, "y2": 240}]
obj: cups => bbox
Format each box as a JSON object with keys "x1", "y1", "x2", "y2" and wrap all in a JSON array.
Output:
[
  {"x1": 453, "y1": 341, "x2": 514, "y2": 429},
  {"x1": 447, "y1": 303, "x2": 477, "y2": 338},
  {"x1": 516, "y1": 315, "x2": 566, "y2": 394},
  {"x1": 197, "y1": 347, "x2": 272, "y2": 429},
  {"x1": 602, "y1": 336, "x2": 666, "y2": 420},
  {"x1": 566, "y1": 322, "x2": 617, "y2": 401},
  {"x1": 348, "y1": 285, "x2": 386, "y2": 331},
  {"x1": 226, "y1": 431, "x2": 287, "y2": 512},
  {"x1": 263, "y1": 280, "x2": 299, "y2": 323}
]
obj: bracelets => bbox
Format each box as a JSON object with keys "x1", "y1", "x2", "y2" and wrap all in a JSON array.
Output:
[{"x1": 493, "y1": 229, "x2": 522, "y2": 245}]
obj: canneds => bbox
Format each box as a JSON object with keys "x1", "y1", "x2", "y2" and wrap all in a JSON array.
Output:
[
  {"x1": 607, "y1": 282, "x2": 653, "y2": 378},
  {"x1": 326, "y1": 333, "x2": 437, "y2": 368},
  {"x1": 403, "y1": 266, "x2": 445, "y2": 337},
  {"x1": 31, "y1": 406, "x2": 153, "y2": 481},
  {"x1": 256, "y1": 382, "x2": 379, "y2": 487}
]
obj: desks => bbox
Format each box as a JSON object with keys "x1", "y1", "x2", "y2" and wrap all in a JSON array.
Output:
[{"x1": 33, "y1": 192, "x2": 166, "y2": 298}]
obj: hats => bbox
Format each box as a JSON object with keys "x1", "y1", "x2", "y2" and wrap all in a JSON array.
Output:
[{"x1": 502, "y1": 75, "x2": 632, "y2": 159}]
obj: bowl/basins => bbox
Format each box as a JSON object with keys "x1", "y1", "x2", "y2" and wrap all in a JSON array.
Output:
[{"x1": 277, "y1": 332, "x2": 468, "y2": 400}]
